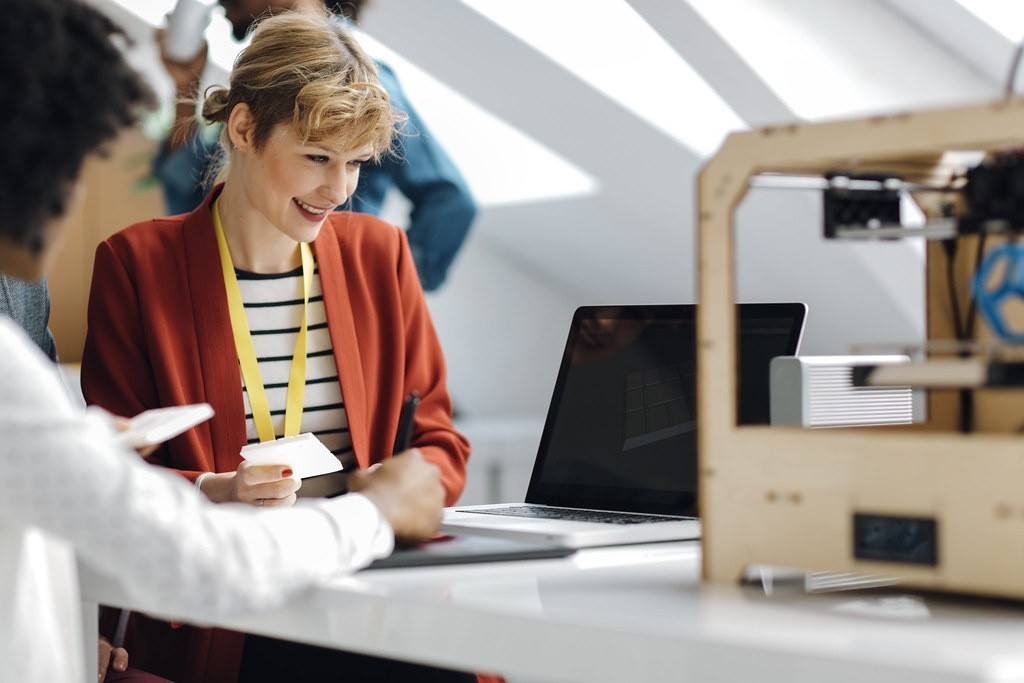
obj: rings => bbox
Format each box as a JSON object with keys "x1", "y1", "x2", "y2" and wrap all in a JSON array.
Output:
[{"x1": 259, "y1": 499, "x2": 265, "y2": 507}]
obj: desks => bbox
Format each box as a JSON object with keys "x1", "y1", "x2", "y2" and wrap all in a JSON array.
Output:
[{"x1": 96, "y1": 503, "x2": 1024, "y2": 683}]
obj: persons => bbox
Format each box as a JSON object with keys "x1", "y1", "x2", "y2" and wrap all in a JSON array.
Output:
[
  {"x1": 151, "y1": 0, "x2": 479, "y2": 293},
  {"x1": 80, "y1": 12, "x2": 505, "y2": 683},
  {"x1": 0, "y1": 0, "x2": 447, "y2": 683}
]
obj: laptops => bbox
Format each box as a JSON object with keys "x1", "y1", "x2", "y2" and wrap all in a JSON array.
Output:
[{"x1": 439, "y1": 303, "x2": 809, "y2": 549}]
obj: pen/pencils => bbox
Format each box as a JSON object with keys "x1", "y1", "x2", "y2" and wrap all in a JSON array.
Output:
[{"x1": 391, "y1": 390, "x2": 419, "y2": 455}]
